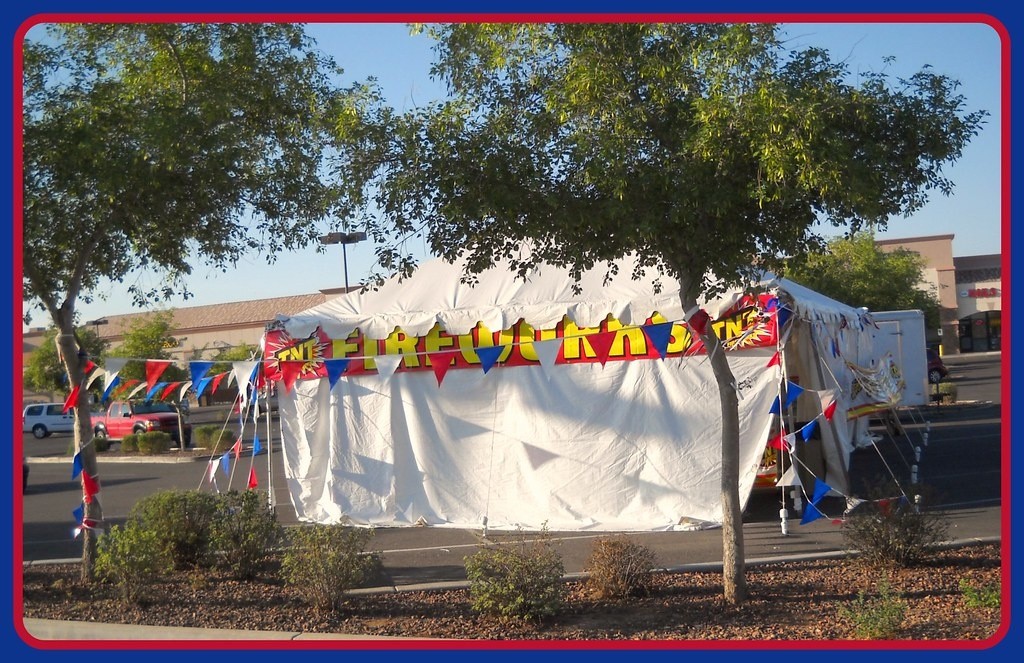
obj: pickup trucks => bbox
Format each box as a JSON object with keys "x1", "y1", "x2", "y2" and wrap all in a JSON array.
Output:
[{"x1": 90, "y1": 398, "x2": 192, "y2": 452}]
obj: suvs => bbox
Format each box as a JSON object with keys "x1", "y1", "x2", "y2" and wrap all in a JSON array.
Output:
[{"x1": 22, "y1": 402, "x2": 103, "y2": 439}]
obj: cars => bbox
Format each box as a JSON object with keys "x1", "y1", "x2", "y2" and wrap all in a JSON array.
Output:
[
  {"x1": 242, "y1": 388, "x2": 278, "y2": 417},
  {"x1": 926, "y1": 347, "x2": 947, "y2": 384}
]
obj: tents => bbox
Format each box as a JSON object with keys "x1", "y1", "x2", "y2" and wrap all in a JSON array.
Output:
[{"x1": 263, "y1": 221, "x2": 875, "y2": 532}]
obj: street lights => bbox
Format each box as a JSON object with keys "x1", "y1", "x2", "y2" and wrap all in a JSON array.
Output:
[
  {"x1": 85, "y1": 319, "x2": 108, "y2": 338},
  {"x1": 318, "y1": 231, "x2": 368, "y2": 294}
]
